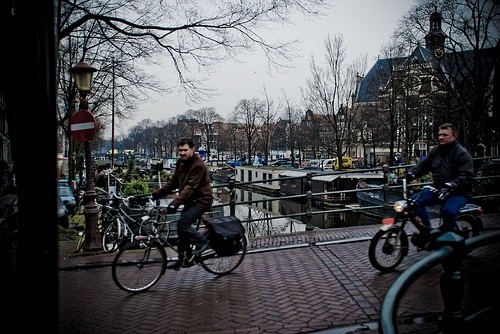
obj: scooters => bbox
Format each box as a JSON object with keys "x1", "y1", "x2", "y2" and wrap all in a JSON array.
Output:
[{"x1": 368, "y1": 171, "x2": 485, "y2": 274}]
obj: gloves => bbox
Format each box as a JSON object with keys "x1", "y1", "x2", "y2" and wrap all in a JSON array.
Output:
[
  {"x1": 145, "y1": 195, "x2": 155, "y2": 203},
  {"x1": 167, "y1": 204, "x2": 177, "y2": 214},
  {"x1": 436, "y1": 183, "x2": 455, "y2": 202},
  {"x1": 396, "y1": 173, "x2": 413, "y2": 185}
]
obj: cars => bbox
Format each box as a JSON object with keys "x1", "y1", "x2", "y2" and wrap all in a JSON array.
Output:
[
  {"x1": 56, "y1": 180, "x2": 77, "y2": 194},
  {"x1": 56, "y1": 186, "x2": 77, "y2": 212},
  {"x1": 56, "y1": 172, "x2": 87, "y2": 188},
  {"x1": 210, "y1": 149, "x2": 404, "y2": 171},
  {"x1": 7, "y1": 195, "x2": 69, "y2": 230}
]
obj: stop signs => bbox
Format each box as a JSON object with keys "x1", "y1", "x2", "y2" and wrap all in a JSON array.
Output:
[{"x1": 69, "y1": 109, "x2": 98, "y2": 143}]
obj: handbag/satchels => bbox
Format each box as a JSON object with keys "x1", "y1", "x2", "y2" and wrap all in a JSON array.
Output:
[{"x1": 205, "y1": 215, "x2": 245, "y2": 258}]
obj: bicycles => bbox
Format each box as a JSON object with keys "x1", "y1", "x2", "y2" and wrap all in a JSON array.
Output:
[
  {"x1": 112, "y1": 198, "x2": 248, "y2": 294},
  {"x1": 74, "y1": 187, "x2": 170, "y2": 254}
]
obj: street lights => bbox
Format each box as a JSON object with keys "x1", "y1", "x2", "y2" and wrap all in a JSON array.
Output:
[{"x1": 72, "y1": 61, "x2": 106, "y2": 257}]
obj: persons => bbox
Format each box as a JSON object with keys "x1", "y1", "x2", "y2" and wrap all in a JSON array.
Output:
[
  {"x1": 151, "y1": 137, "x2": 214, "y2": 269},
  {"x1": 397, "y1": 123, "x2": 475, "y2": 234}
]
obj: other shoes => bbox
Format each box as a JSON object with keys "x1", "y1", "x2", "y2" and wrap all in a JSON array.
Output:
[
  {"x1": 191, "y1": 240, "x2": 210, "y2": 254},
  {"x1": 166, "y1": 260, "x2": 182, "y2": 270}
]
